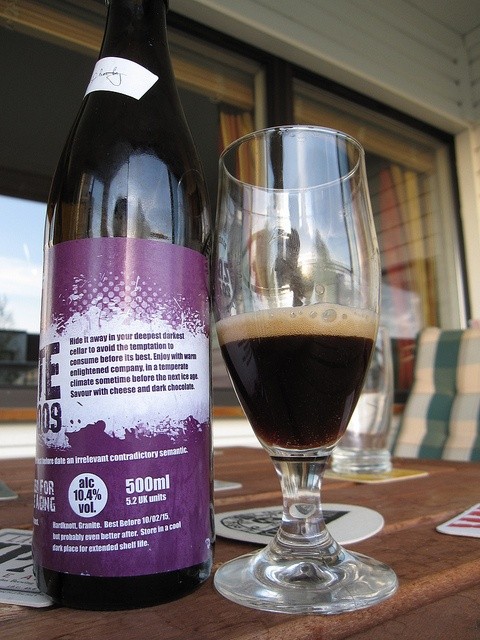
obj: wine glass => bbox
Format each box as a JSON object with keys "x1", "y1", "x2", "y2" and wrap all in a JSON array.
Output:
[{"x1": 209, "y1": 123, "x2": 401, "y2": 619}]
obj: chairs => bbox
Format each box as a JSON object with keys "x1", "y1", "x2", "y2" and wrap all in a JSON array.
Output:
[{"x1": 393, "y1": 327, "x2": 480, "y2": 465}]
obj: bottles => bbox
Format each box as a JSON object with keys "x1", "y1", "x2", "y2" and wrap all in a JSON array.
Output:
[{"x1": 30, "y1": 0, "x2": 219, "y2": 612}]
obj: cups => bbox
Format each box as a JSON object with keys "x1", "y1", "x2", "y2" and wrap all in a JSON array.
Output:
[{"x1": 329, "y1": 320, "x2": 396, "y2": 475}]
